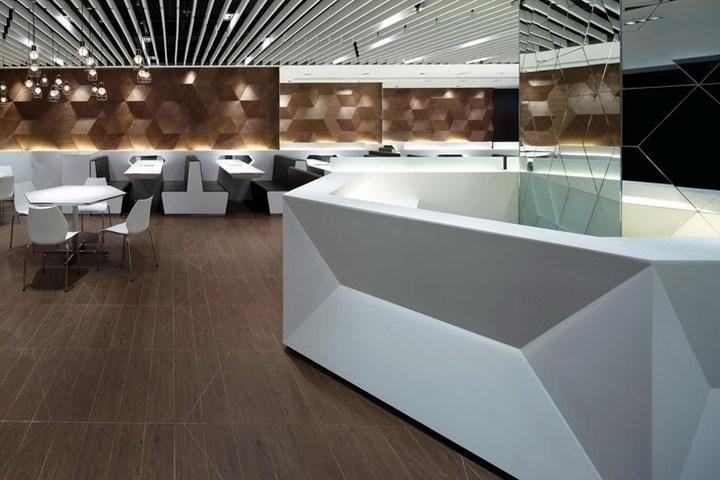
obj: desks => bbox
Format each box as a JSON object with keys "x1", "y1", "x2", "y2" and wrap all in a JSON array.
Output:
[{"x1": 306, "y1": 158, "x2": 334, "y2": 176}]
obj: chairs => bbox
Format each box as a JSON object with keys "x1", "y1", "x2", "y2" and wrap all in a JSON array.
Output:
[{"x1": 0, "y1": 165, "x2": 158, "y2": 293}]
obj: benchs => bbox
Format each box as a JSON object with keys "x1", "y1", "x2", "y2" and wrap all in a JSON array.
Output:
[{"x1": 89, "y1": 155, "x2": 307, "y2": 216}]
